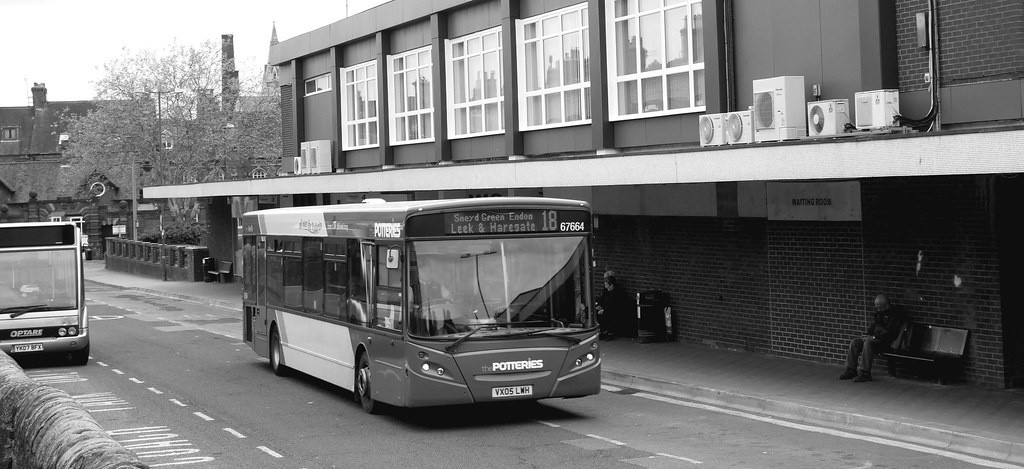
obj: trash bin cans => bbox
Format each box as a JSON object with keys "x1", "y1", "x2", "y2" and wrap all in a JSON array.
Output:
[
  {"x1": 202, "y1": 257, "x2": 216, "y2": 283},
  {"x1": 635, "y1": 288, "x2": 672, "y2": 344}
]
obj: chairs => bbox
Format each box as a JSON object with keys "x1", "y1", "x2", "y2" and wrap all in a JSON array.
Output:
[
  {"x1": 284, "y1": 285, "x2": 303, "y2": 310},
  {"x1": 323, "y1": 293, "x2": 343, "y2": 316},
  {"x1": 304, "y1": 289, "x2": 324, "y2": 316}
]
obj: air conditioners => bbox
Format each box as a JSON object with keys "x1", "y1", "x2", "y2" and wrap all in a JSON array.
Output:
[
  {"x1": 310, "y1": 140, "x2": 332, "y2": 175},
  {"x1": 753, "y1": 75, "x2": 806, "y2": 144},
  {"x1": 855, "y1": 89, "x2": 900, "y2": 131},
  {"x1": 294, "y1": 157, "x2": 301, "y2": 175},
  {"x1": 727, "y1": 111, "x2": 755, "y2": 146},
  {"x1": 698, "y1": 113, "x2": 728, "y2": 147},
  {"x1": 806, "y1": 99, "x2": 850, "y2": 137},
  {"x1": 300, "y1": 142, "x2": 313, "y2": 175}
]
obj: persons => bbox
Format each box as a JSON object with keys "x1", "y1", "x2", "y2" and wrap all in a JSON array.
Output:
[
  {"x1": 406, "y1": 263, "x2": 453, "y2": 317},
  {"x1": 595, "y1": 271, "x2": 616, "y2": 323},
  {"x1": 837, "y1": 294, "x2": 905, "y2": 383},
  {"x1": 598, "y1": 276, "x2": 631, "y2": 341}
]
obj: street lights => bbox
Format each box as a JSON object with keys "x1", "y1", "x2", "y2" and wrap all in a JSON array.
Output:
[{"x1": 132, "y1": 86, "x2": 185, "y2": 283}]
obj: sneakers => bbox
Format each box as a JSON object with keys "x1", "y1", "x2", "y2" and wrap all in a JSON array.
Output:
[
  {"x1": 853, "y1": 371, "x2": 872, "y2": 382},
  {"x1": 839, "y1": 369, "x2": 859, "y2": 380}
]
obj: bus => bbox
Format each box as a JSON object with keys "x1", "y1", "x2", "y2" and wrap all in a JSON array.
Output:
[
  {"x1": 238, "y1": 195, "x2": 605, "y2": 416},
  {"x1": 0, "y1": 215, "x2": 91, "y2": 366}
]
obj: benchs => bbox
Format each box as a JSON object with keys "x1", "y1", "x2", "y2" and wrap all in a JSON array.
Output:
[
  {"x1": 877, "y1": 322, "x2": 970, "y2": 384},
  {"x1": 208, "y1": 260, "x2": 232, "y2": 283}
]
